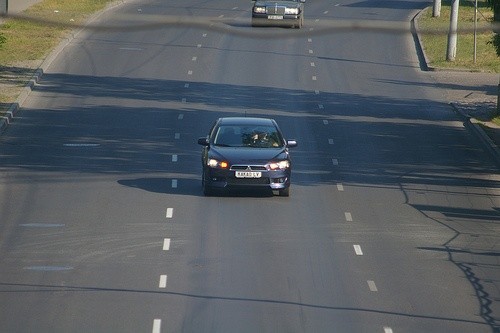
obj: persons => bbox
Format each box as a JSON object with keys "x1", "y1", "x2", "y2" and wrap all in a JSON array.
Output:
[{"x1": 257, "y1": 129, "x2": 268, "y2": 140}]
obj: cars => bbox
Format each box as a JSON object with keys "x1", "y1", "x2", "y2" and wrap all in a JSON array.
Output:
[
  {"x1": 252, "y1": 0, "x2": 307, "y2": 30},
  {"x1": 197, "y1": 117, "x2": 299, "y2": 196}
]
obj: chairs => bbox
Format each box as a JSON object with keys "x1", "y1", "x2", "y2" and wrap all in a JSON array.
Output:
[{"x1": 221, "y1": 128, "x2": 237, "y2": 144}]
